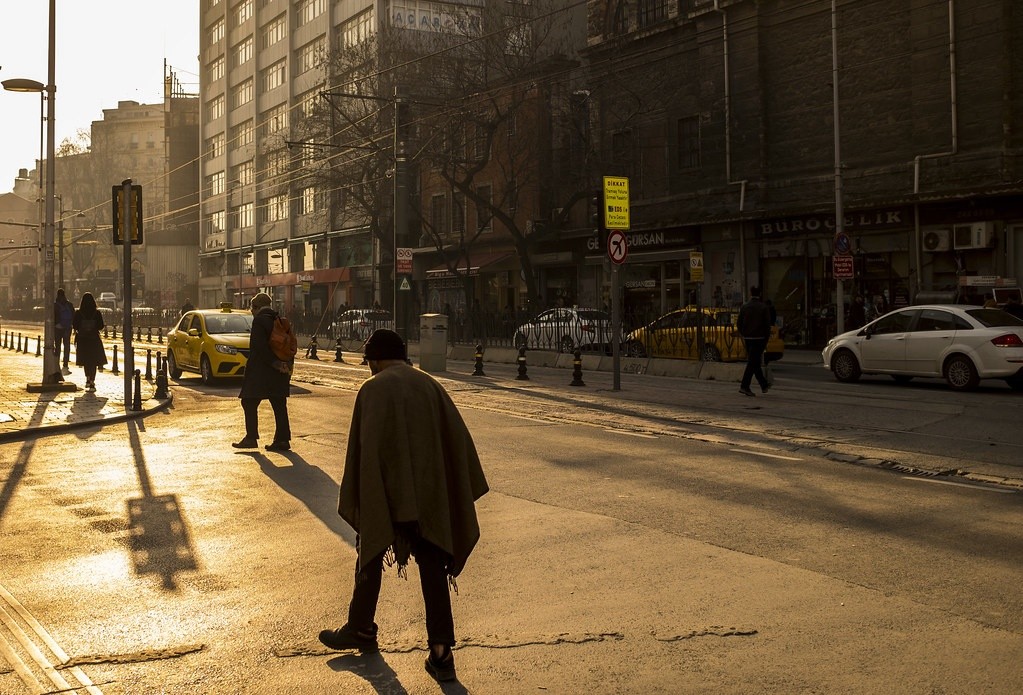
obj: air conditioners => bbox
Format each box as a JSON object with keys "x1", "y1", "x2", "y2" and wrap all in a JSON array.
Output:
[
  {"x1": 953, "y1": 221, "x2": 994, "y2": 249},
  {"x1": 923, "y1": 231, "x2": 953, "y2": 251}
]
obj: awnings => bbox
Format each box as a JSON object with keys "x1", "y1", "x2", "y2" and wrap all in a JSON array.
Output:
[{"x1": 425, "y1": 252, "x2": 515, "y2": 279}]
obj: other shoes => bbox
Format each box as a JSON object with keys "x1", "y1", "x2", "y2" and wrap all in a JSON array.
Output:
[
  {"x1": 62, "y1": 368, "x2": 70, "y2": 374},
  {"x1": 232, "y1": 436, "x2": 258, "y2": 448},
  {"x1": 265, "y1": 441, "x2": 290, "y2": 450},
  {"x1": 86, "y1": 382, "x2": 95, "y2": 391},
  {"x1": 762, "y1": 384, "x2": 771, "y2": 393},
  {"x1": 739, "y1": 388, "x2": 755, "y2": 397}
]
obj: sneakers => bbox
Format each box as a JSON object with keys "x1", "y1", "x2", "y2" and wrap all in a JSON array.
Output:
[
  {"x1": 424, "y1": 644, "x2": 455, "y2": 680},
  {"x1": 317, "y1": 621, "x2": 379, "y2": 652}
]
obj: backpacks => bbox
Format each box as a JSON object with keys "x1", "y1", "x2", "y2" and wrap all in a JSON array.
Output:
[
  {"x1": 263, "y1": 313, "x2": 297, "y2": 362},
  {"x1": 57, "y1": 300, "x2": 73, "y2": 326},
  {"x1": 79, "y1": 310, "x2": 98, "y2": 339}
]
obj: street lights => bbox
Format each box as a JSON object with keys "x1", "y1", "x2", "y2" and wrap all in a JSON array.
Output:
[
  {"x1": 59, "y1": 210, "x2": 85, "y2": 289},
  {"x1": 230, "y1": 178, "x2": 242, "y2": 309},
  {"x1": 1, "y1": 78, "x2": 56, "y2": 382}
]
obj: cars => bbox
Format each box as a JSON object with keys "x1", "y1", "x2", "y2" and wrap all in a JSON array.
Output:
[
  {"x1": 327, "y1": 309, "x2": 394, "y2": 341},
  {"x1": 166, "y1": 302, "x2": 255, "y2": 385},
  {"x1": 626, "y1": 305, "x2": 784, "y2": 365},
  {"x1": 512, "y1": 308, "x2": 624, "y2": 355},
  {"x1": 74, "y1": 308, "x2": 179, "y2": 328},
  {"x1": 822, "y1": 305, "x2": 1023, "y2": 392},
  {"x1": 98, "y1": 292, "x2": 118, "y2": 303}
]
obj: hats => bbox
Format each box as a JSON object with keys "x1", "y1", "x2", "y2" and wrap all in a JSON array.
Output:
[
  {"x1": 364, "y1": 329, "x2": 407, "y2": 361},
  {"x1": 251, "y1": 293, "x2": 271, "y2": 307}
]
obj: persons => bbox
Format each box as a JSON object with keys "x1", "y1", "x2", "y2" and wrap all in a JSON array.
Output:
[
  {"x1": 984, "y1": 293, "x2": 1023, "y2": 320},
  {"x1": 54, "y1": 288, "x2": 75, "y2": 373},
  {"x1": 848, "y1": 293, "x2": 878, "y2": 331},
  {"x1": 72, "y1": 292, "x2": 108, "y2": 390},
  {"x1": 445, "y1": 299, "x2": 486, "y2": 345},
  {"x1": 232, "y1": 294, "x2": 294, "y2": 450},
  {"x1": 182, "y1": 298, "x2": 194, "y2": 316},
  {"x1": 737, "y1": 287, "x2": 772, "y2": 397},
  {"x1": 319, "y1": 329, "x2": 490, "y2": 680}
]
